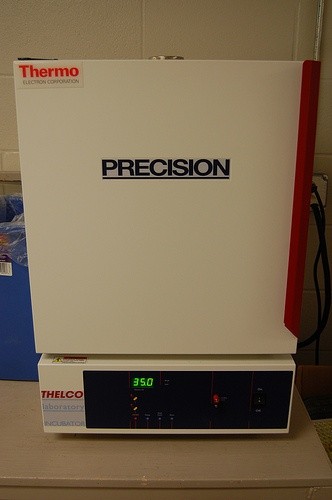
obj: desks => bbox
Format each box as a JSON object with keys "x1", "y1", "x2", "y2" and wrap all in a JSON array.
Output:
[{"x1": 0, "y1": 377, "x2": 332, "y2": 500}]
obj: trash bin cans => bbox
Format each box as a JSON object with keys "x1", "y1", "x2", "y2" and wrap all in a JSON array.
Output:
[{"x1": 0, "y1": 195, "x2": 42, "y2": 381}]
